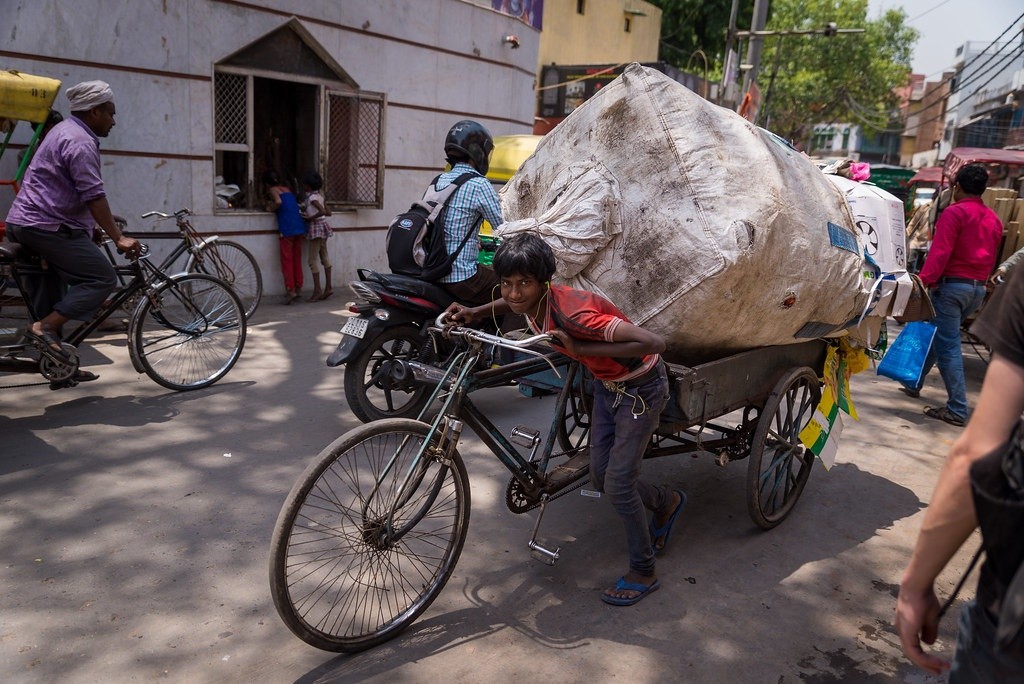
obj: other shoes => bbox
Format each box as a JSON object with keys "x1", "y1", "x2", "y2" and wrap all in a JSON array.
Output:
[{"x1": 282, "y1": 293, "x2": 302, "y2": 305}]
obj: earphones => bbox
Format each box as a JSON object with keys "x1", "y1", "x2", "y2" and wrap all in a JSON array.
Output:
[{"x1": 544, "y1": 281, "x2": 549, "y2": 289}]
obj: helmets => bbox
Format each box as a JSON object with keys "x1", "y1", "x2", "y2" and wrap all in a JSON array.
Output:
[{"x1": 445, "y1": 120, "x2": 495, "y2": 176}]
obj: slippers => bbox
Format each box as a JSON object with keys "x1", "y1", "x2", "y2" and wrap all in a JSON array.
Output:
[
  {"x1": 650, "y1": 489, "x2": 687, "y2": 556},
  {"x1": 49, "y1": 370, "x2": 99, "y2": 390},
  {"x1": 307, "y1": 292, "x2": 334, "y2": 302},
  {"x1": 600, "y1": 576, "x2": 660, "y2": 606},
  {"x1": 24, "y1": 329, "x2": 76, "y2": 366},
  {"x1": 97, "y1": 325, "x2": 129, "y2": 332}
]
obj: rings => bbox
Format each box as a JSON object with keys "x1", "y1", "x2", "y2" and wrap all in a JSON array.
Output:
[{"x1": 132, "y1": 250, "x2": 135, "y2": 253}]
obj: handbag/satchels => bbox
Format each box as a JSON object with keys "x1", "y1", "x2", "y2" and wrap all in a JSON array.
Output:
[{"x1": 877, "y1": 320, "x2": 938, "y2": 391}]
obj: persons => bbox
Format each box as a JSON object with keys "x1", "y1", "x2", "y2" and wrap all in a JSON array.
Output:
[
  {"x1": 905, "y1": 165, "x2": 1004, "y2": 426},
  {"x1": 261, "y1": 170, "x2": 334, "y2": 305},
  {"x1": 422, "y1": 120, "x2": 502, "y2": 305},
  {"x1": 894, "y1": 247, "x2": 1024, "y2": 684},
  {"x1": 5, "y1": 80, "x2": 142, "y2": 382},
  {"x1": 266, "y1": 136, "x2": 289, "y2": 178},
  {"x1": 443, "y1": 233, "x2": 687, "y2": 607}
]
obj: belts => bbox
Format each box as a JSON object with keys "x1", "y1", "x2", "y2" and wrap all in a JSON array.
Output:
[
  {"x1": 598, "y1": 356, "x2": 664, "y2": 393},
  {"x1": 937, "y1": 277, "x2": 986, "y2": 286}
]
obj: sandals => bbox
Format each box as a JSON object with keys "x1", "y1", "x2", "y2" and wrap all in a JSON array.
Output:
[
  {"x1": 898, "y1": 388, "x2": 920, "y2": 398},
  {"x1": 922, "y1": 405, "x2": 965, "y2": 426}
]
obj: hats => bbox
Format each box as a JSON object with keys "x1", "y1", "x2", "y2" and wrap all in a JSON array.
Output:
[{"x1": 65, "y1": 80, "x2": 115, "y2": 111}]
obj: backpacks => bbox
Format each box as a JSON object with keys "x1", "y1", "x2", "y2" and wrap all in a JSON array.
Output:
[
  {"x1": 386, "y1": 173, "x2": 486, "y2": 282},
  {"x1": 937, "y1": 419, "x2": 1024, "y2": 675}
]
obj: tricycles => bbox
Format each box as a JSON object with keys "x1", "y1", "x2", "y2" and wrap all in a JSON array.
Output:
[
  {"x1": 268, "y1": 312, "x2": 858, "y2": 655},
  {"x1": 0, "y1": 70, "x2": 263, "y2": 330},
  {"x1": 0, "y1": 242, "x2": 246, "y2": 390}
]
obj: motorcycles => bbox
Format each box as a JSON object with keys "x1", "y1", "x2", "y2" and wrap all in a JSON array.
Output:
[{"x1": 326, "y1": 268, "x2": 526, "y2": 424}]
